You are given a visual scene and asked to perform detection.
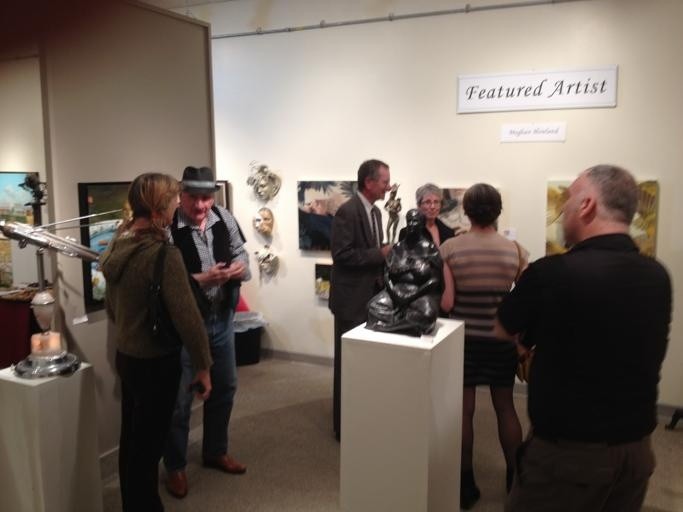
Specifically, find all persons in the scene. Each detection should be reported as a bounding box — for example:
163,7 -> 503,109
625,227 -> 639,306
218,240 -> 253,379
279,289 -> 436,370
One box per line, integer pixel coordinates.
329,159 -> 395,442
399,183 -> 457,249
299,194 -> 352,230
366,209 -> 446,337
494,166 -> 674,512
254,208 -> 273,238
163,166 -> 252,498
246,163 -> 282,200
438,184 -> 536,503
101,173 -> 215,512
254,245 -> 278,274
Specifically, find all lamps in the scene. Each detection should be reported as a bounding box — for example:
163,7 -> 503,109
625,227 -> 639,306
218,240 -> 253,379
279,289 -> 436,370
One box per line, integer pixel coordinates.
16,290 -> 80,378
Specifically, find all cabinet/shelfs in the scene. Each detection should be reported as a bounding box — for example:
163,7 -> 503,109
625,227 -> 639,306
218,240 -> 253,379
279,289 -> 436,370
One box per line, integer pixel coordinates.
340,317 -> 466,511
0,363 -> 102,512
1,288 -> 46,366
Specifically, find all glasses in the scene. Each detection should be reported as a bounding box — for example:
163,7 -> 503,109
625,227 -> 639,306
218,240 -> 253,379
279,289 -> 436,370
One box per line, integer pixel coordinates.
422,200 -> 442,205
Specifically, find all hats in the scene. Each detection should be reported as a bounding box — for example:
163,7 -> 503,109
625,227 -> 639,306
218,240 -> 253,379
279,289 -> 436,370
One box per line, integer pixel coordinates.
177,166 -> 222,194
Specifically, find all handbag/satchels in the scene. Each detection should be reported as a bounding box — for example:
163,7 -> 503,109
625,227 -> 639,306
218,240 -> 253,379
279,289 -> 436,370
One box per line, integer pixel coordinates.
148,241 -> 215,342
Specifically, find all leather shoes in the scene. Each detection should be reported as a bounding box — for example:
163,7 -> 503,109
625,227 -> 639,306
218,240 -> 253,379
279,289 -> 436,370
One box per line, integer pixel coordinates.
463,488 -> 478,511
505,471 -> 514,493
164,461 -> 190,498
202,456 -> 249,475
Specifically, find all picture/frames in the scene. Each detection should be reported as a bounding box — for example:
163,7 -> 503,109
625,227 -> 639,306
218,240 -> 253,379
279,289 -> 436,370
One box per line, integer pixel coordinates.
214,181 -> 229,211
0,172 -> 41,239
544,180 -> 660,267
78,180 -> 178,314
298,180 -> 359,251
